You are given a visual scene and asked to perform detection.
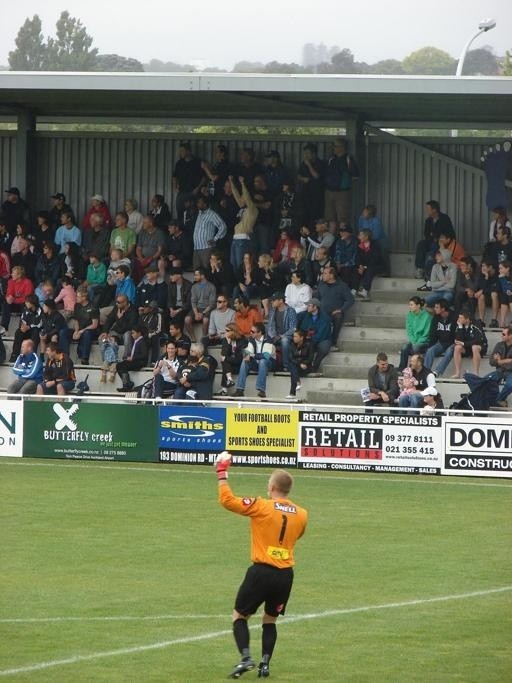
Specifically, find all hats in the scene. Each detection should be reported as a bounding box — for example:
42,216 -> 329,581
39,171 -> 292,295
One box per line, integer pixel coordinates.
263,151 -> 280,158
420,386 -> 438,395
268,291 -> 285,300
304,298 -> 321,307
91,194 -> 104,203
50,192 -> 65,200
141,300 -> 158,309
4,187 -> 20,194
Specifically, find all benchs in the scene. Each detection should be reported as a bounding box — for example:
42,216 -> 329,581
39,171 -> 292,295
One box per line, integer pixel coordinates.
0,240 -> 512,419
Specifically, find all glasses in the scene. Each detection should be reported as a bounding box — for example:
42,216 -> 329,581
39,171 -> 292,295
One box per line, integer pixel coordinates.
216,300 -> 227,303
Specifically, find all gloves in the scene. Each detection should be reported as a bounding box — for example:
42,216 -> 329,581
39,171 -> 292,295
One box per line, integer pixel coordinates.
215,451 -> 233,479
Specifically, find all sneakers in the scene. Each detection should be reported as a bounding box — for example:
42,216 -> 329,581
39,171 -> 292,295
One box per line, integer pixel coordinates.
258,662 -> 269,678
213,380 -> 244,396
417,284 -> 432,290
477,319 -> 485,327
257,390 -> 265,397
286,395 -> 296,399
350,288 -> 357,296
296,386 -> 304,390
117,381 -> 134,392
80,358 -> 88,365
229,657 -> 256,678
100,375 -> 106,382
415,268 -> 424,278
489,319 -> 499,327
109,376 -> 115,382
358,289 -> 368,297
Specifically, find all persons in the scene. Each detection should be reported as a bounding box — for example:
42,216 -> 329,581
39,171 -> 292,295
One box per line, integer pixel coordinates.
1,174 -> 385,400
171,139 -> 360,250
211,450 -> 309,679
365,199 -> 511,413
